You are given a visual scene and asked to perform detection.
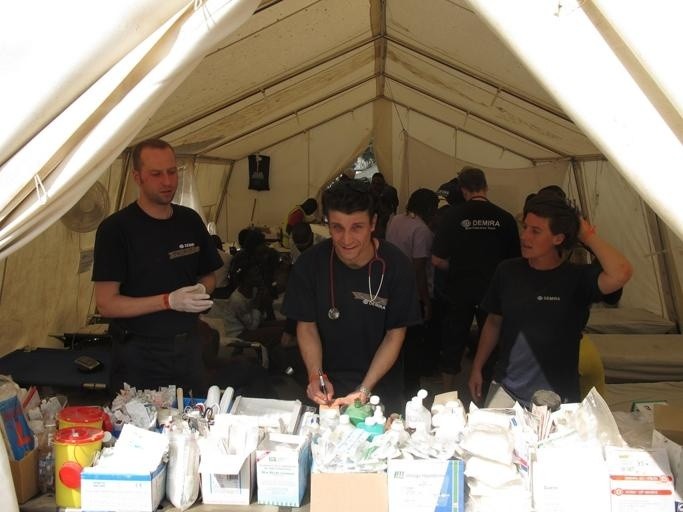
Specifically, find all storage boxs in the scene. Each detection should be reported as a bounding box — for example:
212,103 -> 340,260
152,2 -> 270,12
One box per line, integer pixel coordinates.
312,418 -> 390,512
391,393 -> 464,511
650,403 -> 683,499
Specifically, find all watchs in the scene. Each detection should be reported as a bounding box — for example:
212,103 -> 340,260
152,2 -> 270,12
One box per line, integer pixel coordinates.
357,385 -> 371,396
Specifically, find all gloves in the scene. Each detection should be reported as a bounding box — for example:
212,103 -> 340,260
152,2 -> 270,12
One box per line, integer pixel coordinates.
166,284 -> 213,313
188,283 -> 207,294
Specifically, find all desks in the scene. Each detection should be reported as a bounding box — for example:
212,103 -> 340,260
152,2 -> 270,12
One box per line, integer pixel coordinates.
30,411 -> 680,508
309,223 -> 330,244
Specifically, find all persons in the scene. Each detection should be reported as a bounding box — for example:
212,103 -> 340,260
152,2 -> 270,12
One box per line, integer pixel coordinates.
281,178 -> 422,414
239,232 -> 279,305
340,168 -> 355,182
229,228 -> 288,341
424,178 -> 466,275
209,235 -> 234,299
286,198 -> 327,253
430,168 -> 523,394
369,172 -> 399,238
91,138 -> 224,392
384,188 -> 438,401
470,193 -> 635,408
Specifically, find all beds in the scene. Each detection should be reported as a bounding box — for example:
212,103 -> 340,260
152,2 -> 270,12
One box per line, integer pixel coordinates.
587,307 -> 676,334
586,334 -> 683,380
604,380 -> 683,446
3,340 -> 263,396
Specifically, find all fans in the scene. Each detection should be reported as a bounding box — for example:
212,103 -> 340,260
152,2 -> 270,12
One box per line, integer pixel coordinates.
61,180 -> 110,252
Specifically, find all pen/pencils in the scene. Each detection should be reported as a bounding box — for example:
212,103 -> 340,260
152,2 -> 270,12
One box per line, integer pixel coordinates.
318,368 -> 327,400
